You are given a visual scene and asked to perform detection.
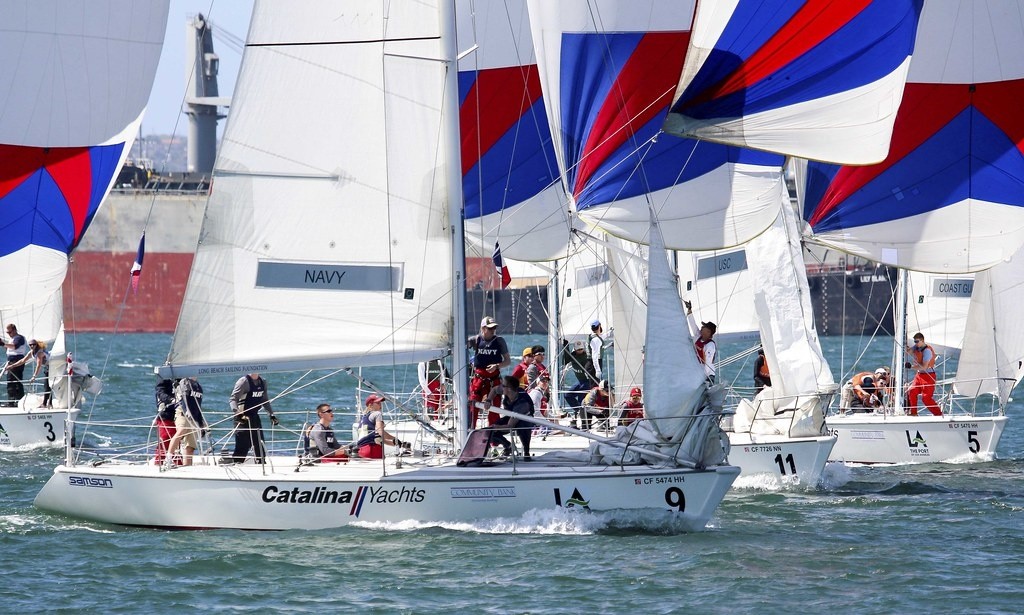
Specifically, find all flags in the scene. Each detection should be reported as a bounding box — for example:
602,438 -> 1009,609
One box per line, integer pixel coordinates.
130,233 -> 145,296
492,243 -> 512,291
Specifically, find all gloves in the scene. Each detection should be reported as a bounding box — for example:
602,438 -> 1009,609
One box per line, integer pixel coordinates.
905,362 -> 911,369
685,301 -> 692,308
270,415 -> 279,426
235,413 -> 247,425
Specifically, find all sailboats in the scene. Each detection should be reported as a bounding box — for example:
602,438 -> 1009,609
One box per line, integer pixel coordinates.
1,0 -> 1024,537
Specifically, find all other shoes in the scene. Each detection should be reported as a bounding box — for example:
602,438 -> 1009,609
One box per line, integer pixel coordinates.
499,446 -> 517,460
524,453 -> 531,462
164,460 -> 175,469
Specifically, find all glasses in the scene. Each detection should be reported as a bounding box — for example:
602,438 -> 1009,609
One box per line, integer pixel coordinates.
323,409 -> 332,414
7,331 -> 12,334
535,352 -> 545,356
29,343 -> 37,346
914,339 -> 923,343
880,382 -> 887,386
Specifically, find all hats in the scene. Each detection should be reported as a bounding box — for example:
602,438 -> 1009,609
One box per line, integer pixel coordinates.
536,376 -> 550,384
701,321 -> 716,334
574,341 -> 584,351
366,395 -> 386,407
599,379 -> 614,392
523,348 -> 532,357
481,316 -> 499,328
875,368 -> 887,374
630,388 -> 642,396
590,320 -> 599,328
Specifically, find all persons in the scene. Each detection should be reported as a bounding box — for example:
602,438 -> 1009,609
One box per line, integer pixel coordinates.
561,340 -> 591,414
466,315 -> 514,448
527,343 -> 551,414
687,302 -> 718,385
618,388 -> 643,426
6,339 -> 54,407
484,373 -> 536,462
418,360 -> 454,420
510,347 -> 533,387
527,376 -> 551,433
161,376 -> 204,466
571,379 -> 611,432
837,333 -> 944,415
586,324 -> 613,387
355,395 -> 411,458
304,401 -> 349,462
154,377 -> 183,465
0,324 -> 27,407
751,344 -> 771,392
227,373 -> 279,464
